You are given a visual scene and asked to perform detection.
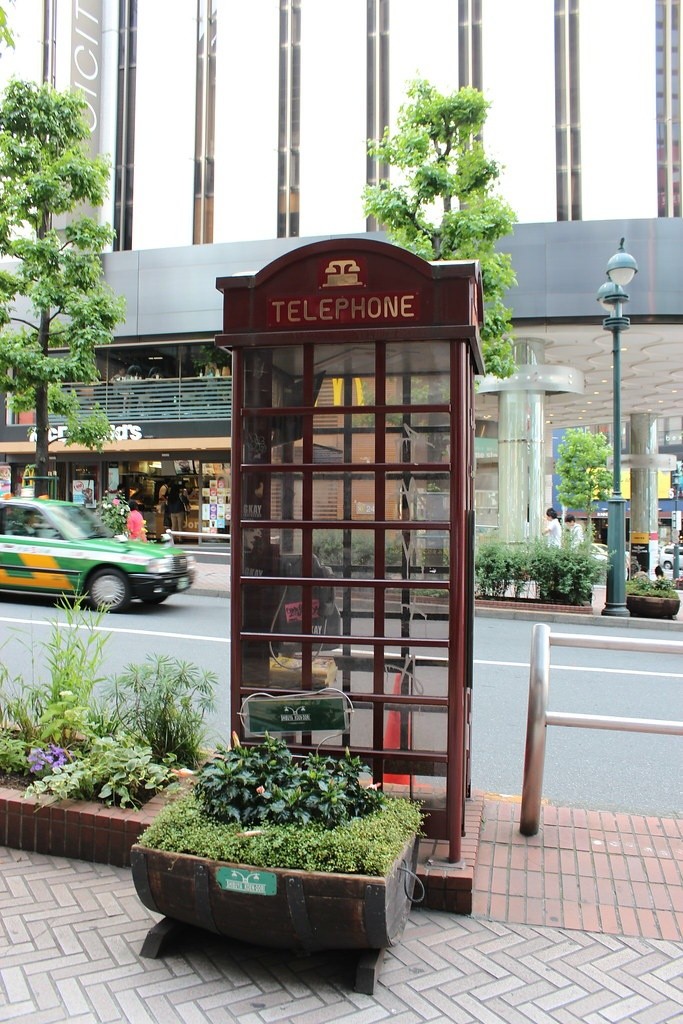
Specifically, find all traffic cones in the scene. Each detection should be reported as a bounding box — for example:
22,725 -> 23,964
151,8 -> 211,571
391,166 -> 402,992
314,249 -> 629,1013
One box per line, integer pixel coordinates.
369,673 -> 434,788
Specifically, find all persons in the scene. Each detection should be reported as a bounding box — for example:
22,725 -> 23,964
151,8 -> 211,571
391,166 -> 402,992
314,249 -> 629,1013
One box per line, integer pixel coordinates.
565,514 -> 583,550
109,367 -> 135,415
128,502 -> 145,541
18,510 -> 38,538
159,476 -> 190,532
542,508 -> 563,548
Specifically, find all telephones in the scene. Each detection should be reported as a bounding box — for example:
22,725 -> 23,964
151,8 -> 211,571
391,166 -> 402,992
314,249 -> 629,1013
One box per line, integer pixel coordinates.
272,555 -> 342,654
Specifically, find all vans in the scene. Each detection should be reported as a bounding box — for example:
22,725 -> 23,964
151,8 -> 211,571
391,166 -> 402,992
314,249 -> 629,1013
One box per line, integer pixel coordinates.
660,546 -> 683,571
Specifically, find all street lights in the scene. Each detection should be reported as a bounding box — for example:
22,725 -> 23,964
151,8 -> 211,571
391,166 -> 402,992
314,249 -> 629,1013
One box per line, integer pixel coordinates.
595,238 -> 639,617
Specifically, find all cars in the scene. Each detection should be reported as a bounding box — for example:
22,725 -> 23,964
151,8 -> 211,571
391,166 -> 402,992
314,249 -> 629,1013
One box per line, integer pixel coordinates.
0,494 -> 198,614
590,543 -> 629,564
